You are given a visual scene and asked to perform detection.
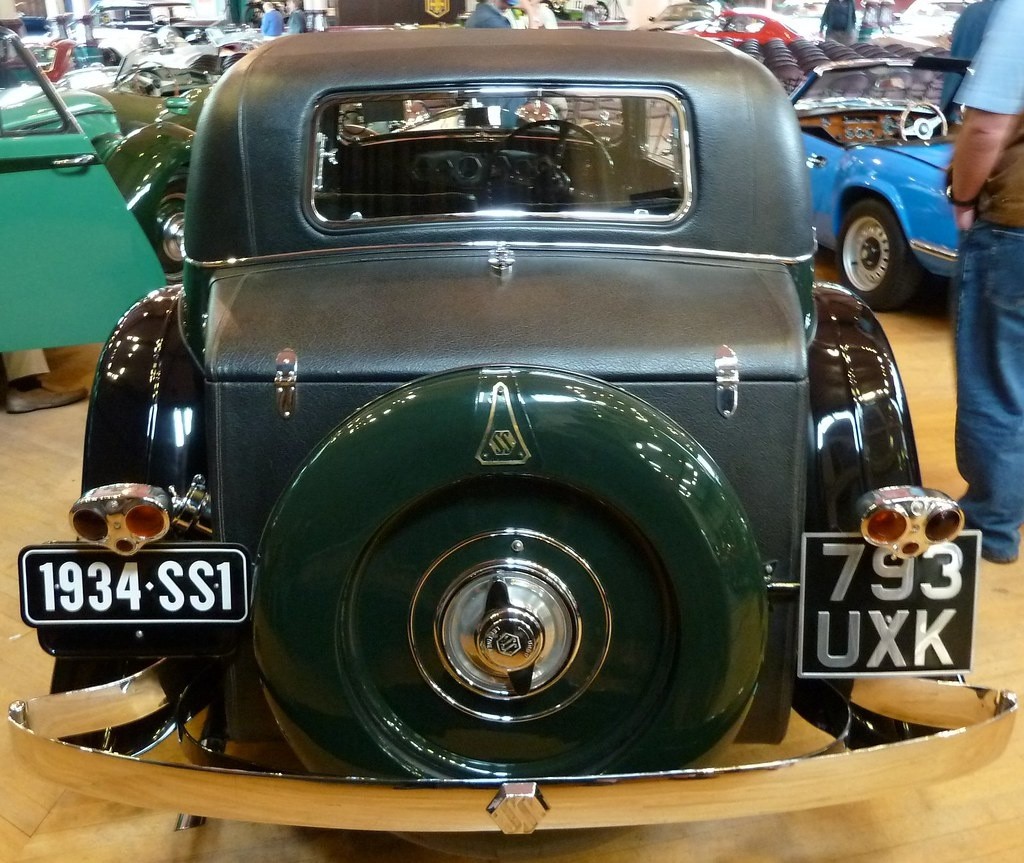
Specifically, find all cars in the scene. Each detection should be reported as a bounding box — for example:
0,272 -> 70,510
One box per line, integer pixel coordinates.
0,0 -> 1024,387
5,26 -> 1024,833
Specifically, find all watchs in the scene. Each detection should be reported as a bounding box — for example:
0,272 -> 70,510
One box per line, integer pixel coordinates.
945,185 -> 975,207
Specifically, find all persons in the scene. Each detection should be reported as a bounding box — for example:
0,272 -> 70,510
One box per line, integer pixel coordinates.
820,0 -> 857,43
262,2 -> 282,36
306,10 -> 328,31
288,0 -> 306,33
52,15 -> 68,39
465,0 -> 558,29
75,15 -> 97,47
940,0 -> 1024,564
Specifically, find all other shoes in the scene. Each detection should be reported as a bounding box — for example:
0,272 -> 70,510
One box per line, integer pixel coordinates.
7,381 -> 88,412
